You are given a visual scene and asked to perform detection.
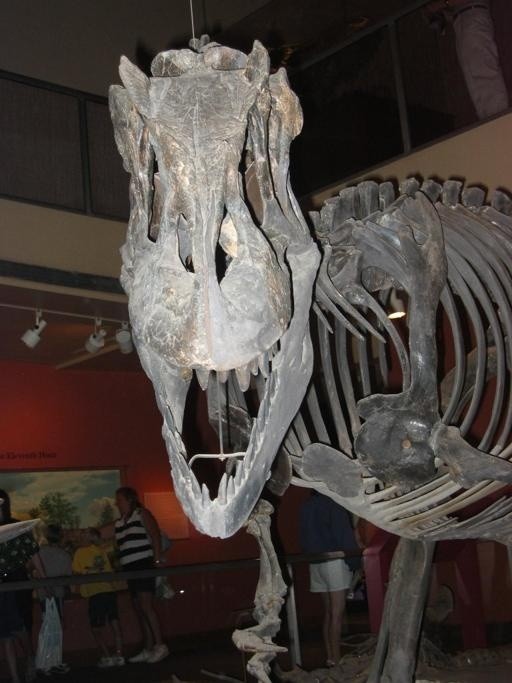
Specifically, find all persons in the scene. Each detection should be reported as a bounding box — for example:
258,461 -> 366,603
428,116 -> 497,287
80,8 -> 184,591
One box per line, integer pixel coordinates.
72,525 -> 126,668
414,0 -> 509,121
1,488 -> 55,682
298,488 -> 367,667
112,486 -> 170,663
32,524 -> 76,677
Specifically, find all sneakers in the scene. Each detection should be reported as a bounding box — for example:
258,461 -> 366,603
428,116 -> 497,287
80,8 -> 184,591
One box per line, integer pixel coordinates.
146,644 -> 170,664
41,667 -> 53,677
98,655 -> 115,668
127,648 -> 152,664
53,662 -> 69,675
112,653 -> 126,666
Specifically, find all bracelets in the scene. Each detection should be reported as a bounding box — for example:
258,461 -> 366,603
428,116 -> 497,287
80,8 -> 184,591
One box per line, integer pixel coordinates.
153,559 -> 160,564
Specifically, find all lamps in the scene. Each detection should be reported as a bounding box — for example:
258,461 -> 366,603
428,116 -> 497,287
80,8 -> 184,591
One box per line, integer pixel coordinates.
82,316 -> 135,357
16,312 -> 52,347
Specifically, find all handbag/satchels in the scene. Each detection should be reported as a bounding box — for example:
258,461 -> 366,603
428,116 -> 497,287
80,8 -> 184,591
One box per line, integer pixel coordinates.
34,596 -> 63,671
140,506 -> 171,552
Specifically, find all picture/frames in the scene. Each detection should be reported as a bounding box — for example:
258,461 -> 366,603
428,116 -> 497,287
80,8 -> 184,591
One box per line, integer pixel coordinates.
0,464 -> 131,602
